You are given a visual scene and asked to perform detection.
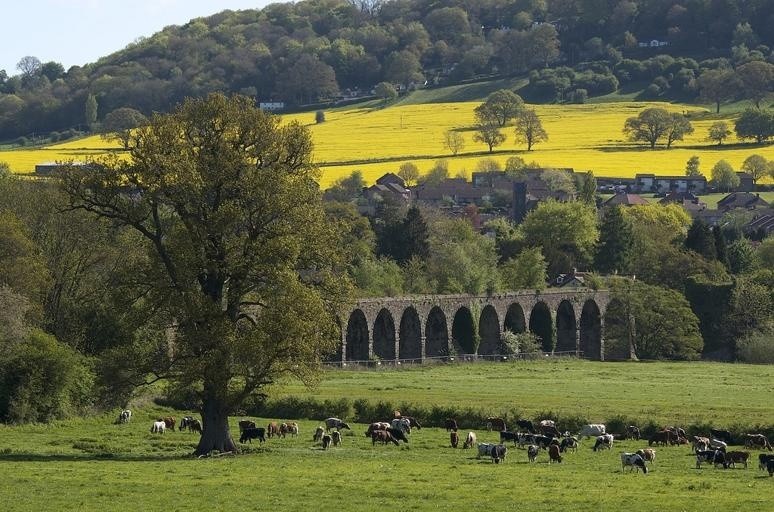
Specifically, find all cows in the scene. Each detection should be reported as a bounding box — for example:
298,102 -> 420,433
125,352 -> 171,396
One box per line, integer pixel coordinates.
445,418 -> 615,464
625,424 -> 640,441
649,426 -> 689,447
313,418 -> 350,450
152,416 -> 202,434
364,408 -> 421,446
619,448 -> 657,474
240,421 -> 299,447
117,410 -> 131,424
692,431 -> 774,476
